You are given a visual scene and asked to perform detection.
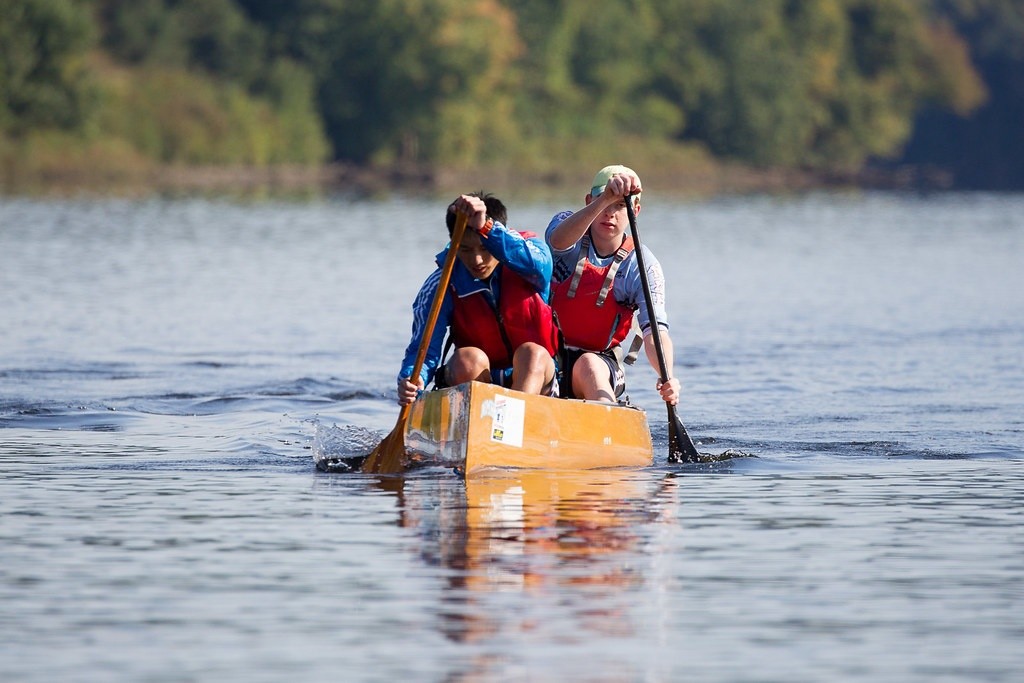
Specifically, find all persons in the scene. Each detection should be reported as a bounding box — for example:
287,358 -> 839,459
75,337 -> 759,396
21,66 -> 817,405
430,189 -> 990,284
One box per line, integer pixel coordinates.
545,165 -> 681,407
398,189 -> 562,399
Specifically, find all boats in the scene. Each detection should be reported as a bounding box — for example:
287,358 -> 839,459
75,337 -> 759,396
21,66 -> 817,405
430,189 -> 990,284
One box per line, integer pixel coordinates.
361,379 -> 654,475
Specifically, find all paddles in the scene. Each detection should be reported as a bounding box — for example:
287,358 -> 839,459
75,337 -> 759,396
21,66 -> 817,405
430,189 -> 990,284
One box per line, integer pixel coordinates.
361,211 -> 470,474
615,184 -> 699,463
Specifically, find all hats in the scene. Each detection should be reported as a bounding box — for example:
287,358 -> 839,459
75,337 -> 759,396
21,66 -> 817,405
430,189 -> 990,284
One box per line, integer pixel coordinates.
591,165 -> 643,202
